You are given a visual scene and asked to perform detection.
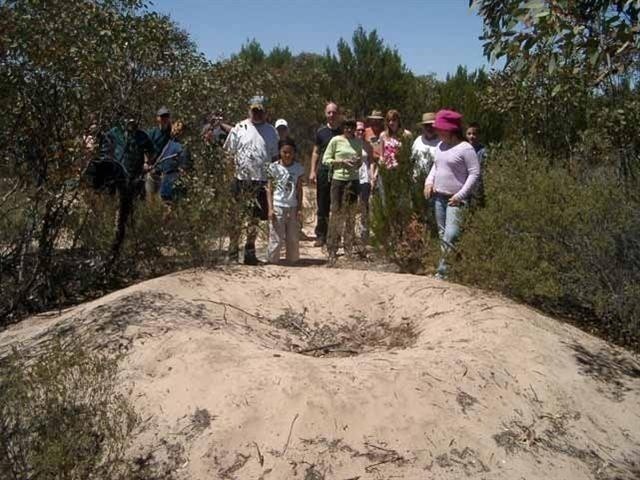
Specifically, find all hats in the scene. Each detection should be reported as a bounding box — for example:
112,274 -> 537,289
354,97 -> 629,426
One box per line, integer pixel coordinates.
432,109 -> 464,131
247,96 -> 271,112
275,119 -> 288,128
416,112 -> 436,125
342,120 -> 356,128
368,110 -> 384,119
157,106 -> 170,116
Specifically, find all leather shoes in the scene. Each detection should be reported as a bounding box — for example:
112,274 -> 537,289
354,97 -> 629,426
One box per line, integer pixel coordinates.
314,240 -> 325,247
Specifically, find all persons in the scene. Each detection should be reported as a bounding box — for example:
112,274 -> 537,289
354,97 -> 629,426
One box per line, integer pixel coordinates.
144,105 -> 172,201
199,109 -> 236,147
411,111 -> 439,239
220,94 -> 280,266
422,109 -> 481,280
306,100 -> 411,261
100,115 -> 153,203
265,139 -> 306,266
464,121 -> 488,208
158,120 -> 191,208
272,118 -> 297,162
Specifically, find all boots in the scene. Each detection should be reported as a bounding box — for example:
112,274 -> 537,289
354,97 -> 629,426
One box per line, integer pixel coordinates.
244,244 -> 264,265
